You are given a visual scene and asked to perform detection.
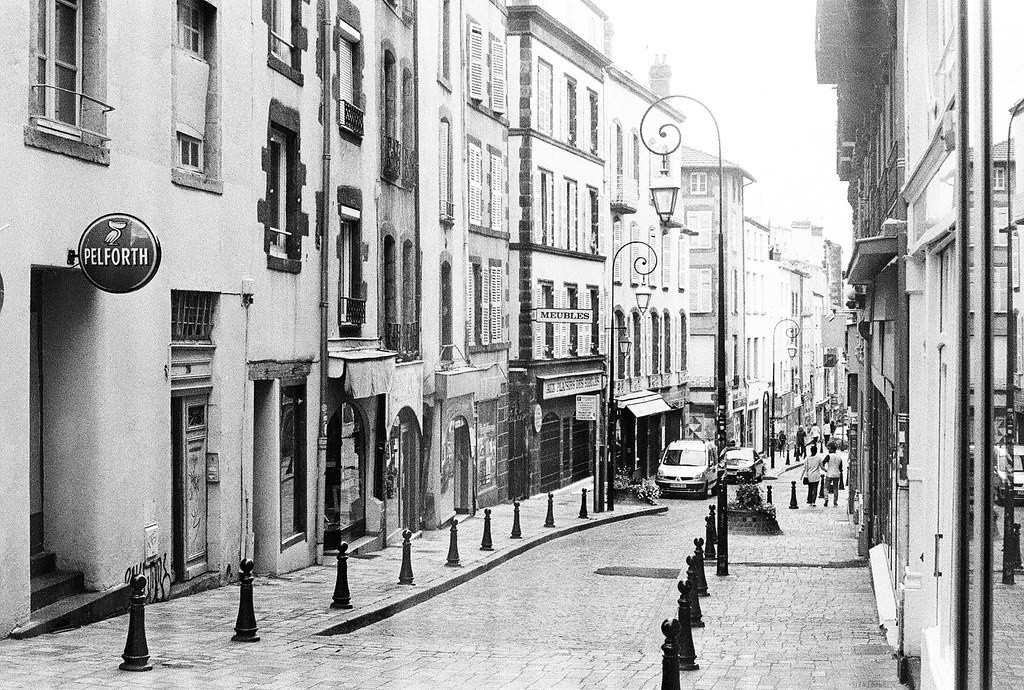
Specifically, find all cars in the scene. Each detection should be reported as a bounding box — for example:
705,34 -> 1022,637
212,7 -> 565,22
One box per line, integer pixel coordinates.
832,427 -> 849,446
721,449 -> 766,484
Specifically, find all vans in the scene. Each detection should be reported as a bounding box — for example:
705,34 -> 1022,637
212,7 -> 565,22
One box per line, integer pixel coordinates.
967,444 -> 1024,508
655,437 -> 720,500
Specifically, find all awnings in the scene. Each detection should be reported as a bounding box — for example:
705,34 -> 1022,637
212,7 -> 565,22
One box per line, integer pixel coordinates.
619,391 -> 672,418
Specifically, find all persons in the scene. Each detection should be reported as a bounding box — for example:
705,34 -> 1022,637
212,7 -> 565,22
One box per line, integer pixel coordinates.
810,423 -> 821,447
830,419 -> 842,434
824,421 -> 830,444
823,445 -> 843,507
797,427 -> 806,456
800,446 -> 827,506
778,430 -> 787,457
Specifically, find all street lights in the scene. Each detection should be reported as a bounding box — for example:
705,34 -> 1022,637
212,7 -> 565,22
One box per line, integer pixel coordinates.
607,240 -> 659,513
639,93 -> 732,578
771,319 -> 801,469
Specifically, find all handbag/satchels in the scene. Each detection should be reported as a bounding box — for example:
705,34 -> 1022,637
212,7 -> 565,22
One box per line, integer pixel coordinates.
802,457 -> 809,485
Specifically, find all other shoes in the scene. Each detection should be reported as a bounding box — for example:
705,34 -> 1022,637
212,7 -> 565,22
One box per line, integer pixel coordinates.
809,503 -> 812,506
833,502 -> 838,506
811,502 -> 815,507
824,499 -> 829,507
